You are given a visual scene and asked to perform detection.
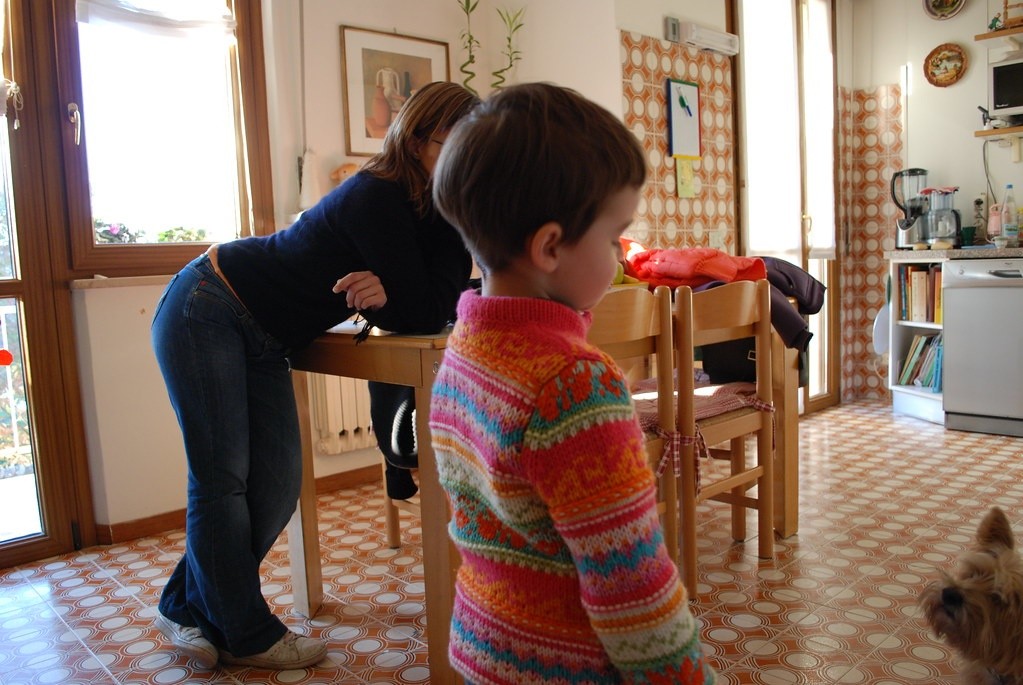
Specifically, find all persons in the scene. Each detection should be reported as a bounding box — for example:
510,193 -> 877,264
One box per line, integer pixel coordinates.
427,82 -> 732,685
149,81 -> 487,669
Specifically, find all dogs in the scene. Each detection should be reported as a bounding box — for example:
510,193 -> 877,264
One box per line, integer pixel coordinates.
915,501 -> 1023,685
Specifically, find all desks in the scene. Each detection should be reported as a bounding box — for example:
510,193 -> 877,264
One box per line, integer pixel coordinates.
291,293 -> 802,619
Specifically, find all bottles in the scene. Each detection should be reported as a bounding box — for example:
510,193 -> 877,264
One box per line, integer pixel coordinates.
1002,184 -> 1018,248
1018,208 -> 1023,242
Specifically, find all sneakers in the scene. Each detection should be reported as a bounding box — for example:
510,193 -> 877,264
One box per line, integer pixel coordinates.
153,614 -> 218,669
219,629 -> 326,670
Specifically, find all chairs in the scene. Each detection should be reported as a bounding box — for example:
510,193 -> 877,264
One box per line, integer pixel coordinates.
581,278 -> 775,599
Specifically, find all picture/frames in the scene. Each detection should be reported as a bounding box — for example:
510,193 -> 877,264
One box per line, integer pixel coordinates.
341,24 -> 451,158
667,78 -> 701,160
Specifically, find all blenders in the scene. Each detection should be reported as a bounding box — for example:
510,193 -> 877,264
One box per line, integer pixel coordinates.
890,168 -> 962,250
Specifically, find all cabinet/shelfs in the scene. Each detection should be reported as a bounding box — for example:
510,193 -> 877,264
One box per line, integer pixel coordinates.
974,26 -> 1023,141
888,260 -> 944,426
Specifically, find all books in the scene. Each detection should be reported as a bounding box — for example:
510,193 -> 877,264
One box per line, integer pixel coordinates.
898,263 -> 942,325
897,333 -> 942,391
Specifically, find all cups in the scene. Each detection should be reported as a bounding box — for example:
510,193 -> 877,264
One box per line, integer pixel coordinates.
991,237 -> 1008,249
962,227 -> 976,246
